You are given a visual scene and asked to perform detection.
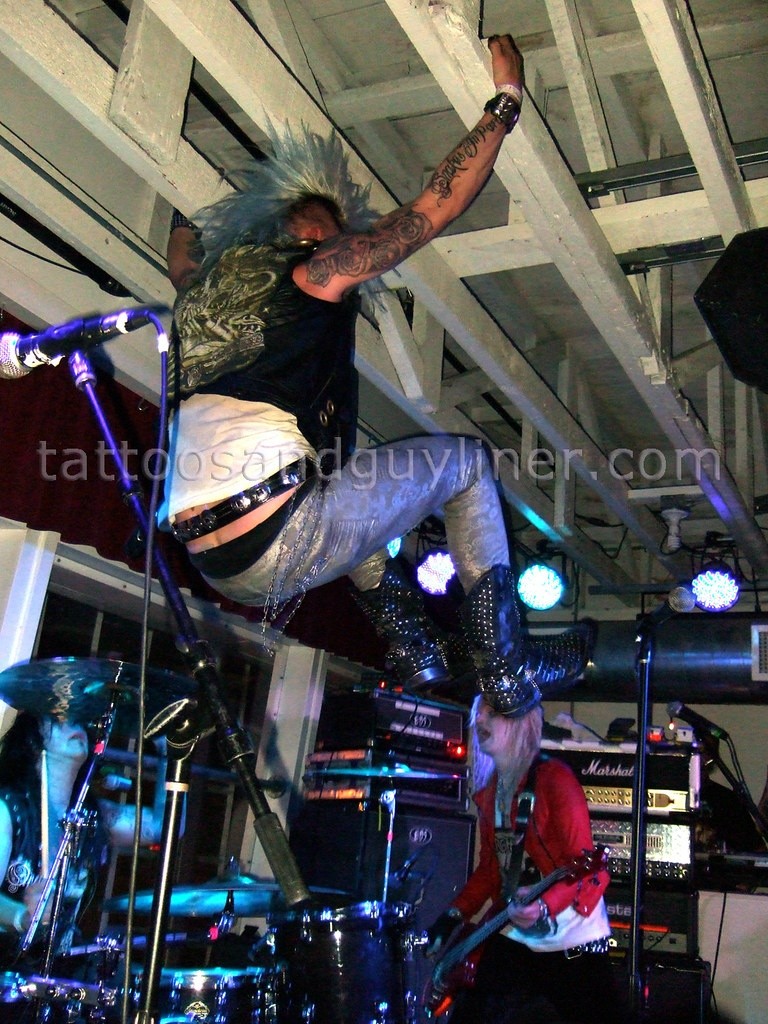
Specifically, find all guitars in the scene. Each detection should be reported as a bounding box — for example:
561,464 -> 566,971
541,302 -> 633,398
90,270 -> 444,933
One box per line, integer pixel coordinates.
419,842 -> 605,1024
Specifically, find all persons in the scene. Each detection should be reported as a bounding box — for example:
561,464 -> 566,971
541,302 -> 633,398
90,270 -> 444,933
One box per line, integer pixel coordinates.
161,33 -> 597,718
0,711 -> 186,976
424,695 -> 611,1024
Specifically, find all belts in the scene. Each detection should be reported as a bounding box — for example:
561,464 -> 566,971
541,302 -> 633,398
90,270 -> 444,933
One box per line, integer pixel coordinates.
171,457 -> 323,544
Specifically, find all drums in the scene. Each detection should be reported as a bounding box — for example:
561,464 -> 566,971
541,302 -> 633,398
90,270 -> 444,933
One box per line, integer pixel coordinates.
125,967 -> 273,1024
266,901 -> 419,1024
0,970 -> 141,1024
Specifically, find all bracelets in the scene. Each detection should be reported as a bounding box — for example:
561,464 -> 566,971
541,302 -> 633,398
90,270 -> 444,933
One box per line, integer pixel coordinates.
484,93 -> 521,135
169,209 -> 203,240
538,898 -> 548,925
444,908 -> 463,921
14,909 -> 27,933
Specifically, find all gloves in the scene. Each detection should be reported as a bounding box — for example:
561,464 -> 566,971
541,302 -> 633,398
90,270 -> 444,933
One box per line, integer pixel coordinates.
424,913 -> 461,958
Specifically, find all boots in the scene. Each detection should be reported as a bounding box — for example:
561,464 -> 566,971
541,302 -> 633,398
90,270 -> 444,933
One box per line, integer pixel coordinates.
349,559 -> 461,690
455,565 -> 598,718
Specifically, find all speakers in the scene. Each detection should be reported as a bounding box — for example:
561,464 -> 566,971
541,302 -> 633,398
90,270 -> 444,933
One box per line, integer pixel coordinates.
298,795 -> 477,1024
611,954 -> 713,1024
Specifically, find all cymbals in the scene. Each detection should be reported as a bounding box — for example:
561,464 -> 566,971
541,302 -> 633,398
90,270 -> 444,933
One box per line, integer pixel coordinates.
98,874 -> 361,918
1,656 -> 198,739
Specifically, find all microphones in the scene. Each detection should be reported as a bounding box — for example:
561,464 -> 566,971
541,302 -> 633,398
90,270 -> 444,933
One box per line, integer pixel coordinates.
0,310 -> 152,379
638,587 -> 695,635
665,701 -> 729,740
